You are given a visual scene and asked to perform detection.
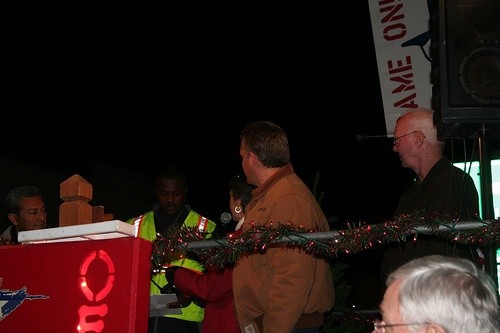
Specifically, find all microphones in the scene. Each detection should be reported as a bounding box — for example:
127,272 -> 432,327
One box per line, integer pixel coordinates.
213,212 -> 232,240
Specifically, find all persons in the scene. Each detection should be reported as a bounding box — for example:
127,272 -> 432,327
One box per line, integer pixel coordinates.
230,120 -> 338,333
165,180 -> 259,333
125,172 -> 221,333
375,255 -> 500,333
2,183 -> 48,243
391,109 -> 482,268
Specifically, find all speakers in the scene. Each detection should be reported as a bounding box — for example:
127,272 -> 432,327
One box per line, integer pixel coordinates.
431,0 -> 500,125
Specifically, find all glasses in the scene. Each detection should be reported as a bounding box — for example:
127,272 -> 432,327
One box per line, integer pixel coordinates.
373,319 -> 431,333
393,130 -> 425,146
